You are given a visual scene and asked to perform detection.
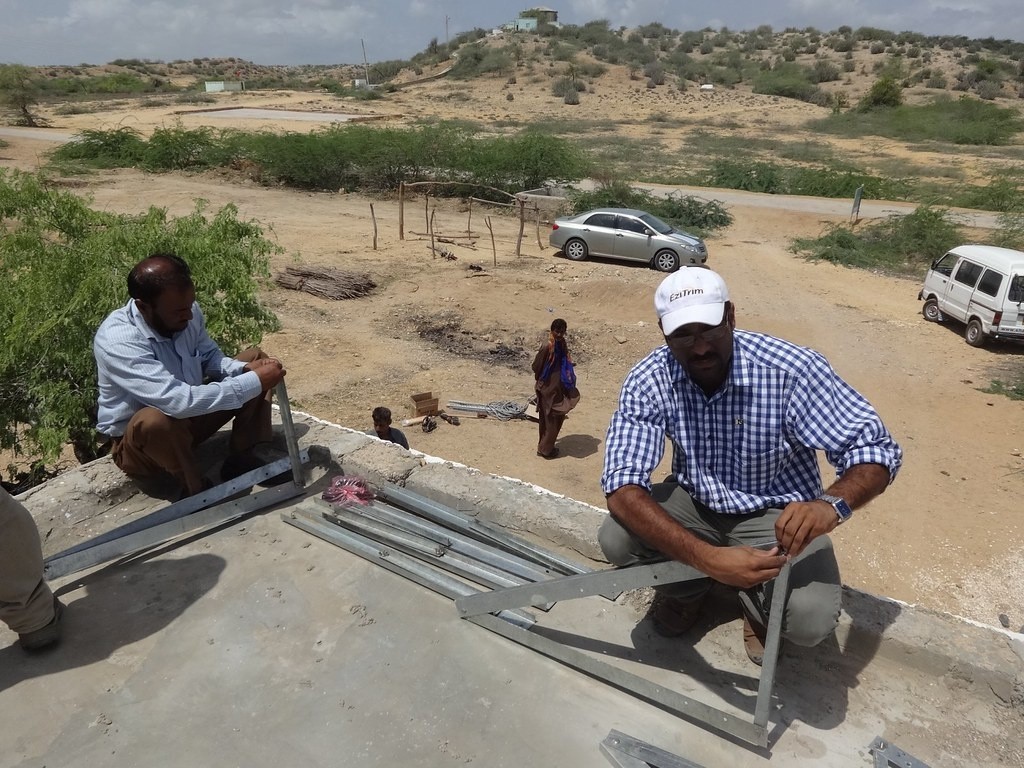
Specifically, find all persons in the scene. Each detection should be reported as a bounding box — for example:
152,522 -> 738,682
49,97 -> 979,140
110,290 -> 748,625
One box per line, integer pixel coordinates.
0,486 -> 63,656
91,253 -> 286,501
598,265 -> 903,666
362,406 -> 410,451
531,318 -> 577,460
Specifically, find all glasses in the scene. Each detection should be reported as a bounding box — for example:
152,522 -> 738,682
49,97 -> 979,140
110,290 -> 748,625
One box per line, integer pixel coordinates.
666,311 -> 729,350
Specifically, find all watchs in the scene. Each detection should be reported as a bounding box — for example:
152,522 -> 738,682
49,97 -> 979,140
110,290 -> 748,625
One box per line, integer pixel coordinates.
816,494 -> 852,526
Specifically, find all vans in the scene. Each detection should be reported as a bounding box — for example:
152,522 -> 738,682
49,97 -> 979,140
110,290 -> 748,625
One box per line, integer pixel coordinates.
919,247 -> 1024,348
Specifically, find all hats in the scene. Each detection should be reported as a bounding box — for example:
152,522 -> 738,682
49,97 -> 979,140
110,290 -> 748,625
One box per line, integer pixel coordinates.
654,266 -> 730,336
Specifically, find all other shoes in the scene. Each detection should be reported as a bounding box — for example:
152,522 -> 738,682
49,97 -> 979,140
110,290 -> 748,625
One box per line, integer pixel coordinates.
744,611 -> 785,666
537,448 -> 559,456
221,457 -> 291,486
655,596 -> 703,637
19,594 -> 64,653
539,449 -> 558,459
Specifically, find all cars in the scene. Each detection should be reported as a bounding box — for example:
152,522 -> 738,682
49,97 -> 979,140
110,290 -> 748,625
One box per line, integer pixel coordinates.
548,209 -> 708,273
